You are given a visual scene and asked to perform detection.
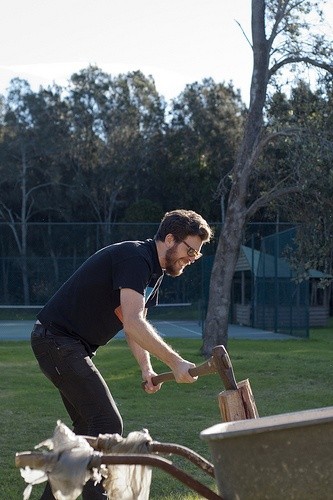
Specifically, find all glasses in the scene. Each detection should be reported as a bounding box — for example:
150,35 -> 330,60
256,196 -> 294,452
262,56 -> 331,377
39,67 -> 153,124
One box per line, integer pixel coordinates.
173,233 -> 203,260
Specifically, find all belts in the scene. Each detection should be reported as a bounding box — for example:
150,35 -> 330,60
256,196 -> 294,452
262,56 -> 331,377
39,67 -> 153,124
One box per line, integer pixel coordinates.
32,324 -> 56,336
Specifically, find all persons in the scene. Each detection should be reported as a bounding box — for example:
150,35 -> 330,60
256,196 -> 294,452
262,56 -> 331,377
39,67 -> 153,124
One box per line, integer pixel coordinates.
29,208 -> 216,500
